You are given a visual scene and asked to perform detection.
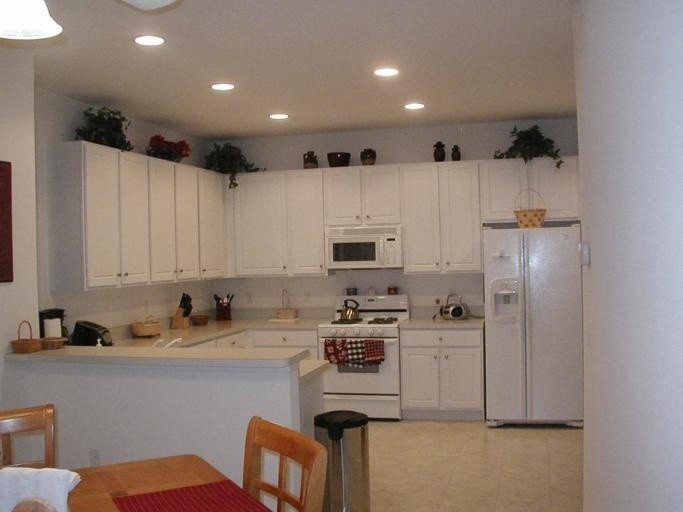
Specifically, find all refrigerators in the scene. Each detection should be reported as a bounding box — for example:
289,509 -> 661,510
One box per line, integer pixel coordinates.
483,224 -> 583,426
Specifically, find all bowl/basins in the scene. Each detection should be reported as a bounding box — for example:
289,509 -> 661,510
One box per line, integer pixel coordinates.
190,315 -> 209,327
327,152 -> 351,166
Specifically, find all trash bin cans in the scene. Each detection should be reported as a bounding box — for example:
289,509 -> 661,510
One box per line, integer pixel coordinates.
312,410 -> 369,512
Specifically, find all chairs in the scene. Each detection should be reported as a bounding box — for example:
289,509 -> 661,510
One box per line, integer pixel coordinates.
0,404 -> 64,469
243,416 -> 332,511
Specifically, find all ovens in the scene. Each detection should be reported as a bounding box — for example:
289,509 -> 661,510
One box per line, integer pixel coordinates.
318,338 -> 400,397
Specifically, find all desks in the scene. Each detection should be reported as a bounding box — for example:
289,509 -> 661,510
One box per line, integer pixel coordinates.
60,454 -> 273,511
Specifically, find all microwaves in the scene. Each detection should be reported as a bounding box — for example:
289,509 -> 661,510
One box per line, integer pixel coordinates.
324,226 -> 402,270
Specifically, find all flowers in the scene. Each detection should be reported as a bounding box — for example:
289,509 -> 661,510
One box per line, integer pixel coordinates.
147,133 -> 193,162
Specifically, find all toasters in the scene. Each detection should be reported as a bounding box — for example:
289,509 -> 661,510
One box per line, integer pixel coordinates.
72,319 -> 113,345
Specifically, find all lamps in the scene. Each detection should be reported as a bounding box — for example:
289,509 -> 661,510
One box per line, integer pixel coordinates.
1,1 -> 66,41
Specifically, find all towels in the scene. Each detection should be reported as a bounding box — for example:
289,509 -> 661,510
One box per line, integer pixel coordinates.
323,336 -> 349,366
0,466 -> 83,511
363,338 -> 385,366
341,340 -> 367,370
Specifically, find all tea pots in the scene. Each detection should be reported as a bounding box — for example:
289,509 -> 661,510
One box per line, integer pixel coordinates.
341,299 -> 360,322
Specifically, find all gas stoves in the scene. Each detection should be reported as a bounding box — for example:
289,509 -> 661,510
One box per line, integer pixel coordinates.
331,316 -> 398,325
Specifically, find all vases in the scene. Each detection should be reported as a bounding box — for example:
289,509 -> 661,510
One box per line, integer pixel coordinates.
145,152 -> 185,163
325,152 -> 353,167
361,148 -> 376,167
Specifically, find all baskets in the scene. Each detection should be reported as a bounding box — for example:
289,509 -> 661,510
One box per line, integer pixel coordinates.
9,321 -> 42,353
131,321 -> 160,336
513,189 -> 546,228
42,336 -> 68,349
278,289 -> 297,319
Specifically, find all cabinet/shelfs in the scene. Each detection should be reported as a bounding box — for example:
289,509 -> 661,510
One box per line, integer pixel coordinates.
320,323 -> 404,425
231,170 -> 285,281
481,156 -> 579,223
175,327 -> 249,351
249,326 -> 319,351
284,167 -> 324,277
326,168 -> 399,225
148,157 -> 199,287
40,139 -> 149,293
399,160 -> 483,276
198,167 -> 233,285
400,318 -> 487,423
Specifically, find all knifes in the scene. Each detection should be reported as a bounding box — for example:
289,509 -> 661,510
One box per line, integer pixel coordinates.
179,293 -> 193,318
